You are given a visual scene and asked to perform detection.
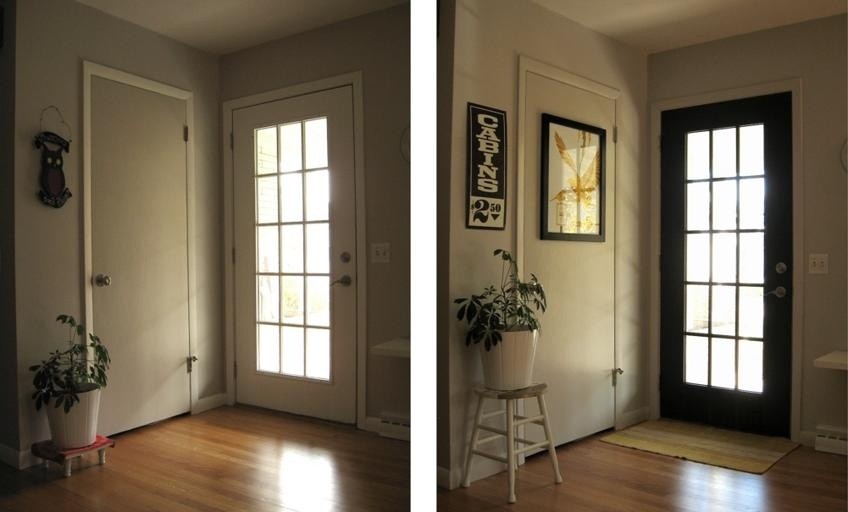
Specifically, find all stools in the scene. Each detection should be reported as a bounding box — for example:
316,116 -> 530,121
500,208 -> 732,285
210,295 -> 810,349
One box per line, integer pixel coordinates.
460,385 -> 563,505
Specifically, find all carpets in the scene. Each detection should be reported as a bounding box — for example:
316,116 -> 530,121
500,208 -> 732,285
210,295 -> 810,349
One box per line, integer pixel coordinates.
596,416 -> 802,475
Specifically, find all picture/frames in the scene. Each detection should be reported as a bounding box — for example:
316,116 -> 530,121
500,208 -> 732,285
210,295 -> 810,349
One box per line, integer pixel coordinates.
537,109 -> 607,243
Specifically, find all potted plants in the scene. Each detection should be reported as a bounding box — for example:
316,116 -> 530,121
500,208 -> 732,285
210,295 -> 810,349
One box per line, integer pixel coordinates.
450,246 -> 549,392
25,312 -> 112,448
